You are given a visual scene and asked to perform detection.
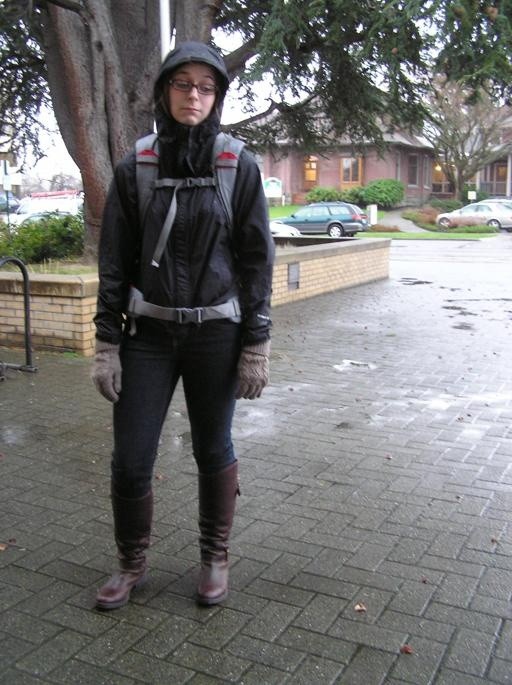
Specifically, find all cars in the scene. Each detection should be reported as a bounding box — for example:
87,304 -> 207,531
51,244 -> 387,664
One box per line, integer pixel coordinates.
270,220 -> 302,237
479,198 -> 512,233
0,192 -> 87,232
436,202 -> 511,231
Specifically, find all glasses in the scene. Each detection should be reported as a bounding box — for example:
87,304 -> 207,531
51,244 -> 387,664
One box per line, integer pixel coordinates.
168,79 -> 219,95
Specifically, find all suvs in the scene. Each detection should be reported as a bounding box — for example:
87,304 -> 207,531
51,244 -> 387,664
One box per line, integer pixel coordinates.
272,199 -> 370,238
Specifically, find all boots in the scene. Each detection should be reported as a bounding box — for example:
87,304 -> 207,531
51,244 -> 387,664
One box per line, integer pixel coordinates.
197,458 -> 240,606
96,480 -> 153,609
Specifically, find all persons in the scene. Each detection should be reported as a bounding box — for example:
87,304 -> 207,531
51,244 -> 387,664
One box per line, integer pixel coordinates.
88,38 -> 280,614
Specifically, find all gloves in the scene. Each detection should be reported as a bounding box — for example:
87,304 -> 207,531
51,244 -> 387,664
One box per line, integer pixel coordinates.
235,339 -> 271,400
92,338 -> 123,404
